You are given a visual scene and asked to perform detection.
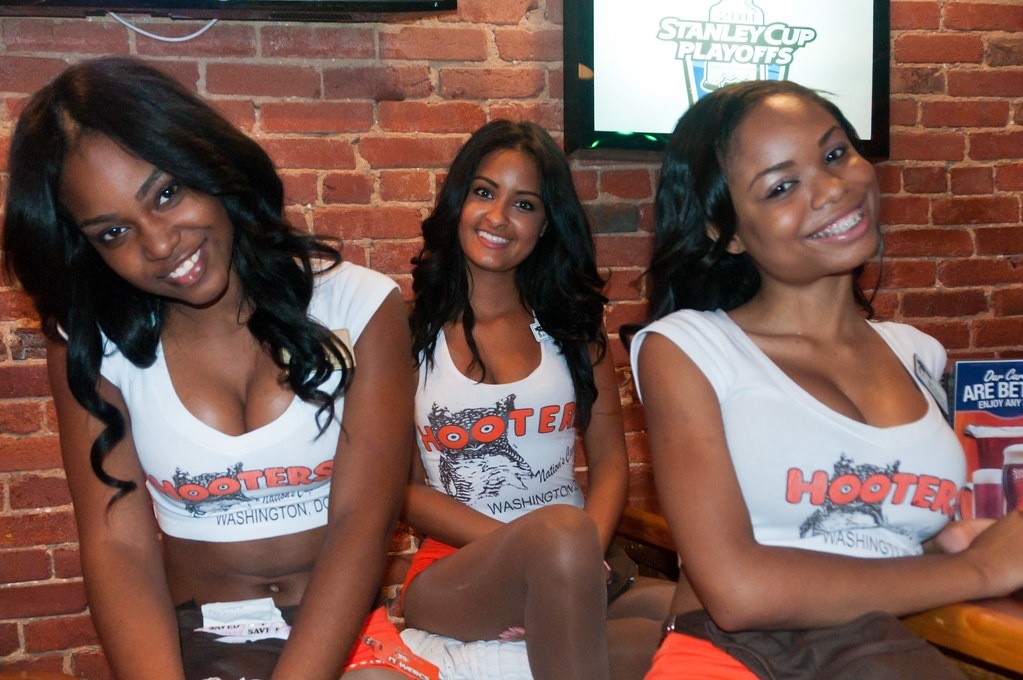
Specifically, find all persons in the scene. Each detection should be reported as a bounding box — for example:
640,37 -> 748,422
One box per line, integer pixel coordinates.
624,79 -> 1023,680
404,117 -> 675,680
0,56 -> 421,680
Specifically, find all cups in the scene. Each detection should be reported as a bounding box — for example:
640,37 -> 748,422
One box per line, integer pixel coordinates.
954,468 -> 1005,521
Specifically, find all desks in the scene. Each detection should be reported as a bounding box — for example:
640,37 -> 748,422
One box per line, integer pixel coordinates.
617,503 -> 1023,680
